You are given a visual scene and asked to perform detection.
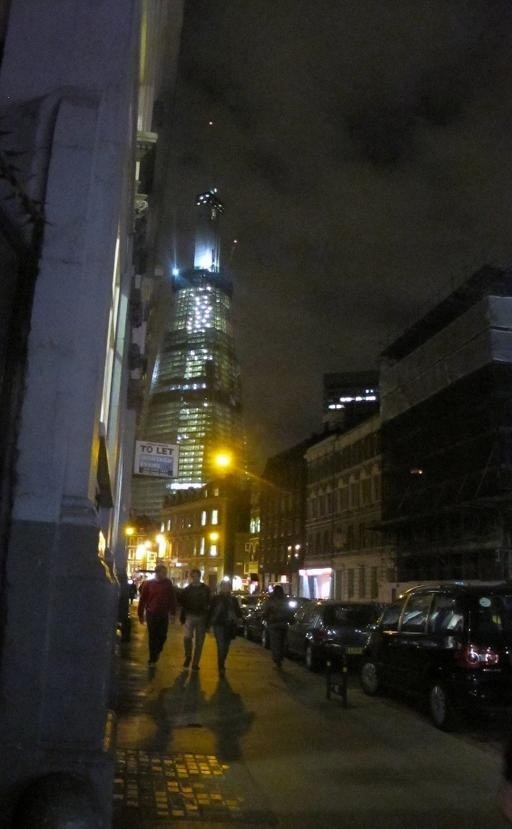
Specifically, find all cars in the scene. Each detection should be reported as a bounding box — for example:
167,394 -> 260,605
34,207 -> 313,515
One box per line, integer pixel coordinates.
287,599 -> 382,671
227,590 -> 312,648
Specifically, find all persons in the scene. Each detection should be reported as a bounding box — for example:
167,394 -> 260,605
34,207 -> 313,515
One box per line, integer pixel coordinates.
210,580 -> 245,678
137,563 -> 175,669
256,584 -> 294,670
176,568 -> 212,670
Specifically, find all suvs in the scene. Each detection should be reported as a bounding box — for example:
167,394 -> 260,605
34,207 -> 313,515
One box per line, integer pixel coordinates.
357,582 -> 510,733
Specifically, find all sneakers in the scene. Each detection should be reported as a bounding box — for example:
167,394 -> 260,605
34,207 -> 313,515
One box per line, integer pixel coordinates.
184,657 -> 201,671
